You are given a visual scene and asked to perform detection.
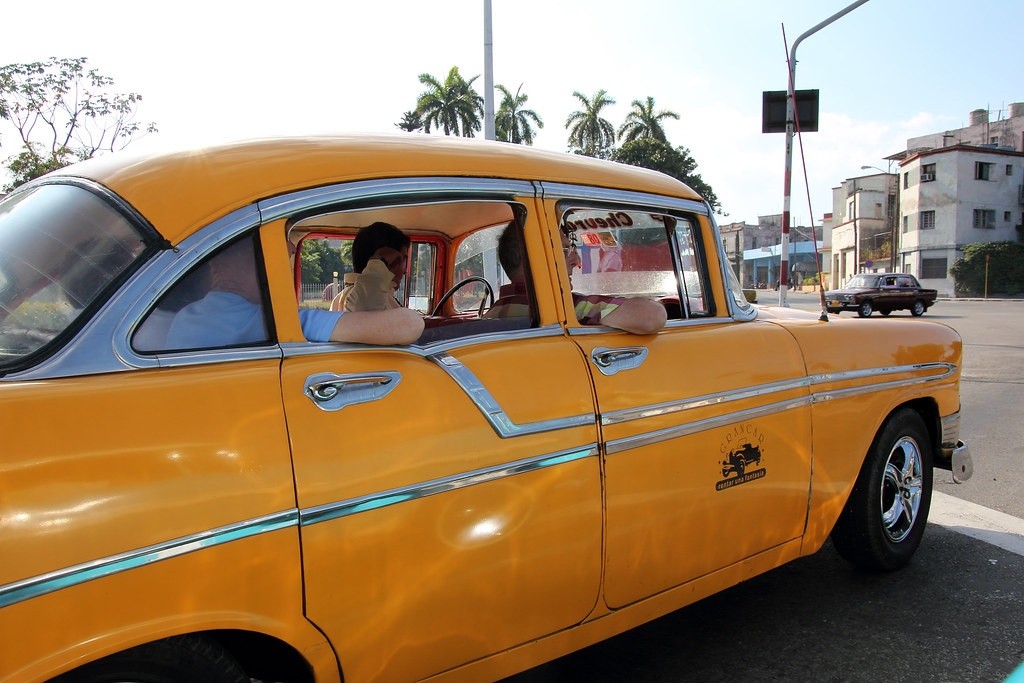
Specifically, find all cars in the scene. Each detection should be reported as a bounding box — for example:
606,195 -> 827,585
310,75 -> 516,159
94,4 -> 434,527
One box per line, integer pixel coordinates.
1,134 -> 973,683
823,273 -> 938,317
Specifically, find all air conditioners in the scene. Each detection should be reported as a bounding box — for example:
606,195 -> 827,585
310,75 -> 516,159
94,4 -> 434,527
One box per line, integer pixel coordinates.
920,174 -> 932,181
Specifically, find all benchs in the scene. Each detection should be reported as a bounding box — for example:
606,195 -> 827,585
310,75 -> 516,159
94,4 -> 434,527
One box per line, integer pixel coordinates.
417,315 -> 531,346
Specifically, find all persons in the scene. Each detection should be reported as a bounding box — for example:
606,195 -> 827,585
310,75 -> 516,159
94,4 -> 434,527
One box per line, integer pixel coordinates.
61,219 -> 425,352
330,222 -> 411,310
480,217 -> 667,335
